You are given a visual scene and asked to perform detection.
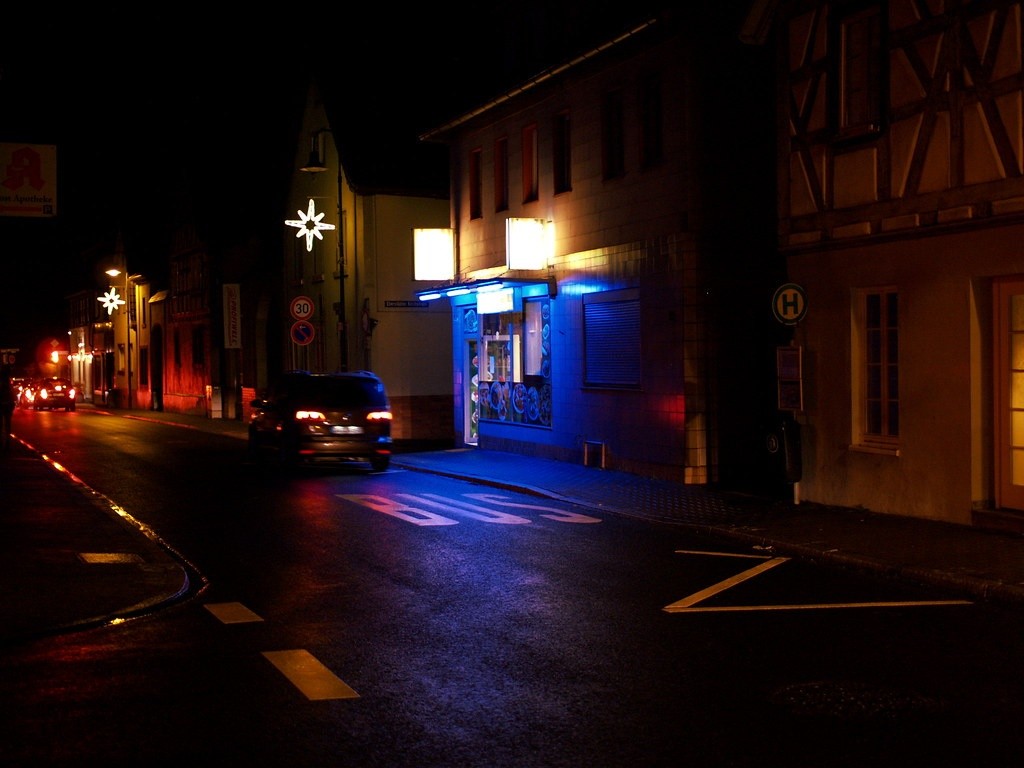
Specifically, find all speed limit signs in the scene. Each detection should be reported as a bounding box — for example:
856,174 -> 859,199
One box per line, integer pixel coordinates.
290,296 -> 315,321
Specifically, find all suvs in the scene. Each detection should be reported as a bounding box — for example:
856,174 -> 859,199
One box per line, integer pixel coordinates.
247,369 -> 394,476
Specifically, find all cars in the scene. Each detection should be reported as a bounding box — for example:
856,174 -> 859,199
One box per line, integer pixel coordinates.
10,378 -> 77,411
